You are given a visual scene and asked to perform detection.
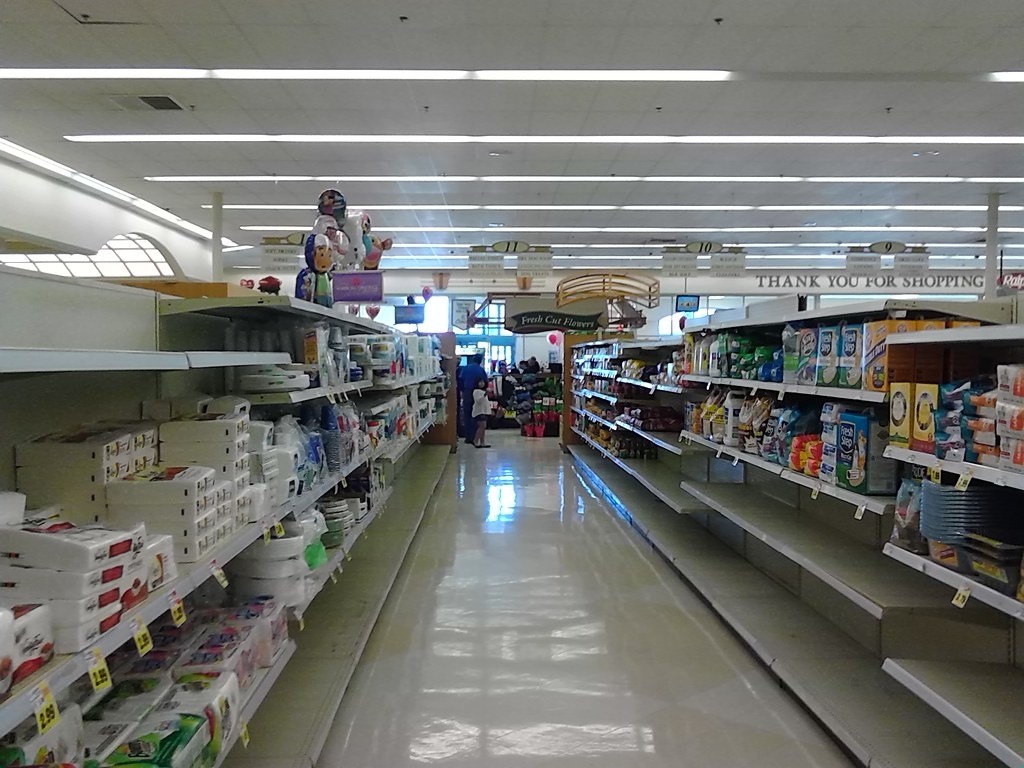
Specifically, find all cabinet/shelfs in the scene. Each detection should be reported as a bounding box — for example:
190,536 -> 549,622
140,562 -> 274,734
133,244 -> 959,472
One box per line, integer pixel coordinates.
157,293 -> 447,622
674,298 -> 1013,666
881,323 -> 1024,768
570,338 -> 746,534
0,265 -> 296,768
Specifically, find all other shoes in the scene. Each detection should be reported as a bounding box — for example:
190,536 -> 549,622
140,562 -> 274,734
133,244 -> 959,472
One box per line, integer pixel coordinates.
471,440 -> 480,448
464,439 -> 472,443
480,444 -> 491,448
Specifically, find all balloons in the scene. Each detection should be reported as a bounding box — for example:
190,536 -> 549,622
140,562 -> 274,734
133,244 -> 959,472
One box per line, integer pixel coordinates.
547,330 -> 563,348
238,187 -> 395,324
422,286 -> 433,304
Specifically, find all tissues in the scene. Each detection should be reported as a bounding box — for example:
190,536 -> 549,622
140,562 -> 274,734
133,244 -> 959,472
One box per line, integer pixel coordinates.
0,391 -> 287,768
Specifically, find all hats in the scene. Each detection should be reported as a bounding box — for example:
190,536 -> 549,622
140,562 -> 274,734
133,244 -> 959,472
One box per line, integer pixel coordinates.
473,354 -> 482,362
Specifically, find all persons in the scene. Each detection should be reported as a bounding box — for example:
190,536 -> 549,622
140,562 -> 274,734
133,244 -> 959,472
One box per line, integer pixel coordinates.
529,356 -> 539,374
498,360 -> 508,373
457,353 -> 488,444
456,355 -> 461,437
519,361 -> 536,374
471,378 -> 491,448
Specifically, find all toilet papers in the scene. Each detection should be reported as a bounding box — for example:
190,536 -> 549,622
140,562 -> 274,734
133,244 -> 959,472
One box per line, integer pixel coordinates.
224,320 -> 450,609
